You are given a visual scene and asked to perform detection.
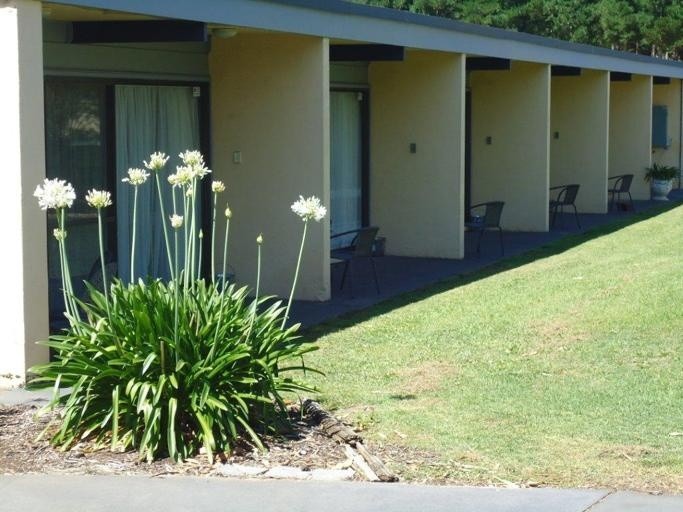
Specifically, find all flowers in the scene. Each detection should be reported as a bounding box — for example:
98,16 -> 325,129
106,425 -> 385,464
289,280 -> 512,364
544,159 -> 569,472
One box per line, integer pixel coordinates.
23,147 -> 327,465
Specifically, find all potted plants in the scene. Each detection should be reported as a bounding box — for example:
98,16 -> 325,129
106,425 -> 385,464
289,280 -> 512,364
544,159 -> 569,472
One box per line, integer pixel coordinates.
641,163 -> 682,202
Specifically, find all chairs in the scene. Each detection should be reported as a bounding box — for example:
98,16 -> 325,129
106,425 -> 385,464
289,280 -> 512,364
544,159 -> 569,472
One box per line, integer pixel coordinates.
464,201 -> 507,258
608,173 -> 634,211
549,184 -> 582,230
330,225 -> 381,295
